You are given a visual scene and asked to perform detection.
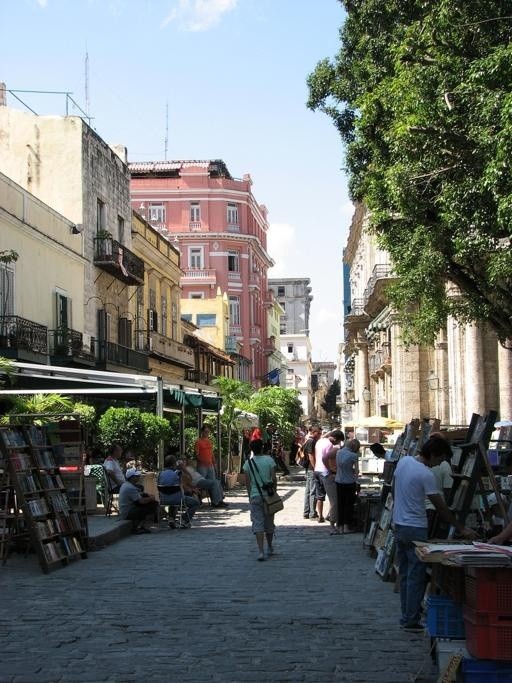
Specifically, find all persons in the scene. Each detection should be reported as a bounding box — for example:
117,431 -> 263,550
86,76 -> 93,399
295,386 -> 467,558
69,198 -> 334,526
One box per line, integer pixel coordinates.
392,434 -> 480,633
194,426 -> 216,479
118,468 -> 158,534
182,453 -> 229,508
102,445 -> 144,493
156,454 -> 200,529
265,421 -> 360,535
242,439 -> 278,561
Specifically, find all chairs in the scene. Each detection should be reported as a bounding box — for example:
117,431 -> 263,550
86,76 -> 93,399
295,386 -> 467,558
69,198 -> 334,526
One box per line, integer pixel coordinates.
156,484 -> 191,532
99,463 -> 121,517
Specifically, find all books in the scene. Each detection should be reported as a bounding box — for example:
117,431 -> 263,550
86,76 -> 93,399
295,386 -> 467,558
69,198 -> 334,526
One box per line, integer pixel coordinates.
0,427 -> 83,563
366,421 -> 512,577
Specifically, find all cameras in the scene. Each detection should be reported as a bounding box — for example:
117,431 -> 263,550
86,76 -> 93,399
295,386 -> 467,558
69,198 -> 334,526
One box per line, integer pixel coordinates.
262,483 -> 275,496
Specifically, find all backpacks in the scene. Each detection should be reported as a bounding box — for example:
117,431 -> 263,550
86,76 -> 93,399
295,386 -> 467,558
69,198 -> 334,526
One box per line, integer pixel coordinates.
293,438 -> 312,469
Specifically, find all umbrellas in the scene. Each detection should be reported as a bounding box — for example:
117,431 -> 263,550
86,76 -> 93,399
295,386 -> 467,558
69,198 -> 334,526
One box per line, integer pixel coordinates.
342,414 -> 405,444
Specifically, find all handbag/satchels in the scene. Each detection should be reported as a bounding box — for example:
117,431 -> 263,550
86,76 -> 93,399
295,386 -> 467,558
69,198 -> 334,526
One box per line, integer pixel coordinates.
262,492 -> 284,518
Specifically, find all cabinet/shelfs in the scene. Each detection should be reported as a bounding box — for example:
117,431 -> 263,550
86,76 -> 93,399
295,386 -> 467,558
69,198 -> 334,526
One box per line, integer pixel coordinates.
0,419 -> 87,575
346,409 -> 512,615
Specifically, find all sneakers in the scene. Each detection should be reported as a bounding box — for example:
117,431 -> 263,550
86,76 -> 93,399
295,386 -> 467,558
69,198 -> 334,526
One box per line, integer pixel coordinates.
255,547 -> 274,561
399,621 -> 427,633
211,501 -> 227,507
134,527 -> 152,535
302,512 -> 356,535
168,518 -> 193,529
282,470 -> 290,476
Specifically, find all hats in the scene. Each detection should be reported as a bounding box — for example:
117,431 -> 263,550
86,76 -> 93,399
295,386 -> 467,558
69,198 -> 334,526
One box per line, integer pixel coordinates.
125,467 -> 142,479
